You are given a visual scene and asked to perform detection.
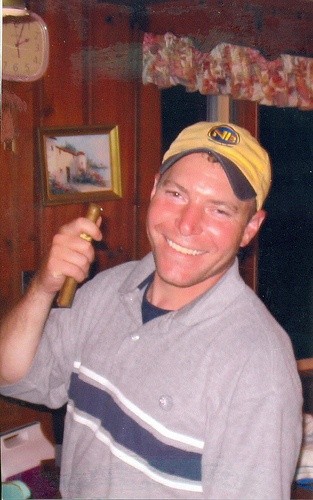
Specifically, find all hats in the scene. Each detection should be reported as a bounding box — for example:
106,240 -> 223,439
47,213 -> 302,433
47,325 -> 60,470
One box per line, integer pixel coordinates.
159,122 -> 274,212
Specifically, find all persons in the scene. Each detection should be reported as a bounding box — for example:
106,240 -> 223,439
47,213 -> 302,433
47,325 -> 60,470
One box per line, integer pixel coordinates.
0,120 -> 306,500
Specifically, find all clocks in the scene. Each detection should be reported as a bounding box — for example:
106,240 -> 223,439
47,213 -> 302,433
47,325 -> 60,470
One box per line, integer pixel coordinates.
2,7 -> 48,81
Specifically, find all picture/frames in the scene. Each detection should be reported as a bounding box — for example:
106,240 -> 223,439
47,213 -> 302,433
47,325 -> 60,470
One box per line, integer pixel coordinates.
34,124 -> 122,206
